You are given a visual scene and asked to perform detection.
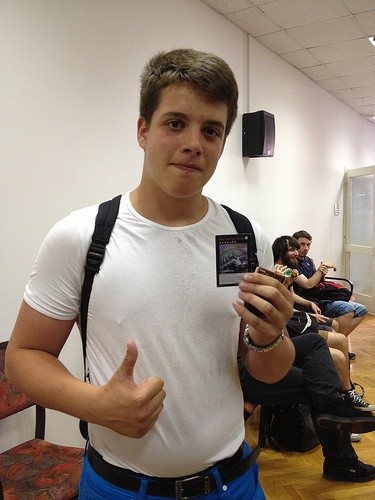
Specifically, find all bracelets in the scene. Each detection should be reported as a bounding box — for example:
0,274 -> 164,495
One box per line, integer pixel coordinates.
242,322 -> 286,354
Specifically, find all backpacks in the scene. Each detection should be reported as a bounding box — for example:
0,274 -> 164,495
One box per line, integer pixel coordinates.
302,280 -> 352,302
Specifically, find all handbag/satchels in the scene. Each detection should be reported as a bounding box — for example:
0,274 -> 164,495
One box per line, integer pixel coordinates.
266,399 -> 320,454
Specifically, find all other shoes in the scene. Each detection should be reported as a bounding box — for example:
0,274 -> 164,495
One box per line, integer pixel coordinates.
348,352 -> 356,360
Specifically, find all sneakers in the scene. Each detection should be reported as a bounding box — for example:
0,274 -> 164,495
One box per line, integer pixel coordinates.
349,433 -> 360,441
323,456 -> 375,483
317,393 -> 375,433
344,380 -> 375,411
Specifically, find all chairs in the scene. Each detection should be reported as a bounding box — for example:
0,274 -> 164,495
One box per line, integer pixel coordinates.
0,340 -> 85,500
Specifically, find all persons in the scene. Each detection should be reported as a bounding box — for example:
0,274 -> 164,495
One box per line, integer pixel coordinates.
4,49 -> 295,500
237,231 -> 375,483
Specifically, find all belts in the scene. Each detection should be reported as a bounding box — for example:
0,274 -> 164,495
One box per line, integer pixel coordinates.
87,442 -> 262,500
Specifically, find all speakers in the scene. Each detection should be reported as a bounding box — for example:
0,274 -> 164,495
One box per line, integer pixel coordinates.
242,110 -> 275,158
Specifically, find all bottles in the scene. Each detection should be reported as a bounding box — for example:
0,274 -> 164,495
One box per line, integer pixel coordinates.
279,265 -> 292,278
